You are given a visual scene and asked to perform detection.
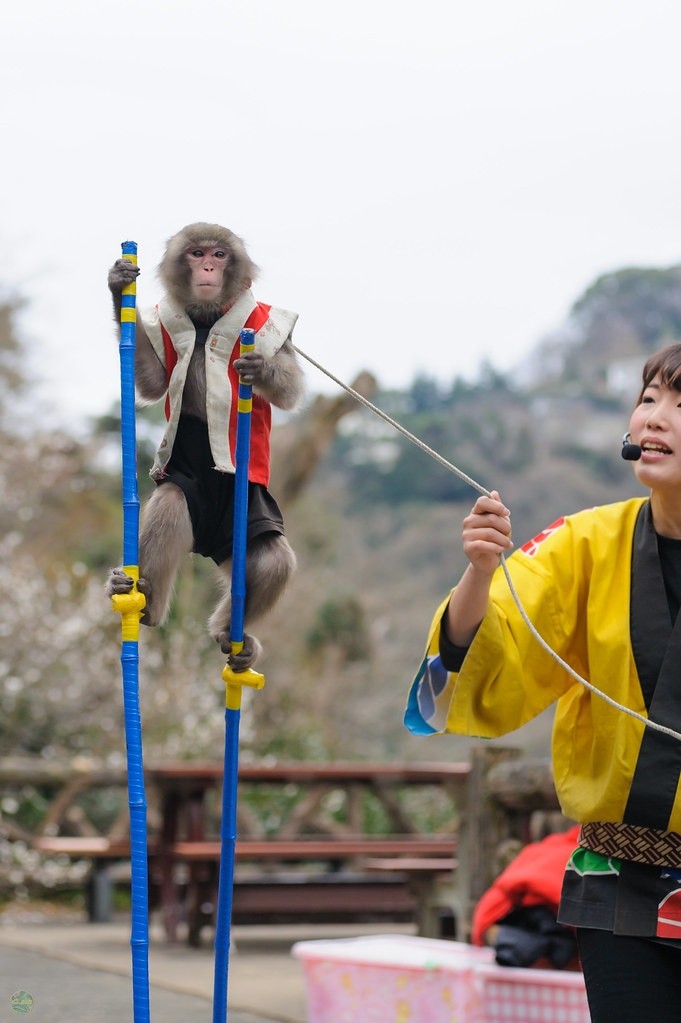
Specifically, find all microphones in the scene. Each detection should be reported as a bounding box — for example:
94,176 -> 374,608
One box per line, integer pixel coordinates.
621,432 -> 642,462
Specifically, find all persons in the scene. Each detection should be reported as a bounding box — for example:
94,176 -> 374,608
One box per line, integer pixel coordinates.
401,344 -> 681,1022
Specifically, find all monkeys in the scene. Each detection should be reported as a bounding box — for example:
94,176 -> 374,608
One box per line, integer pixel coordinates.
102,219 -> 304,676
